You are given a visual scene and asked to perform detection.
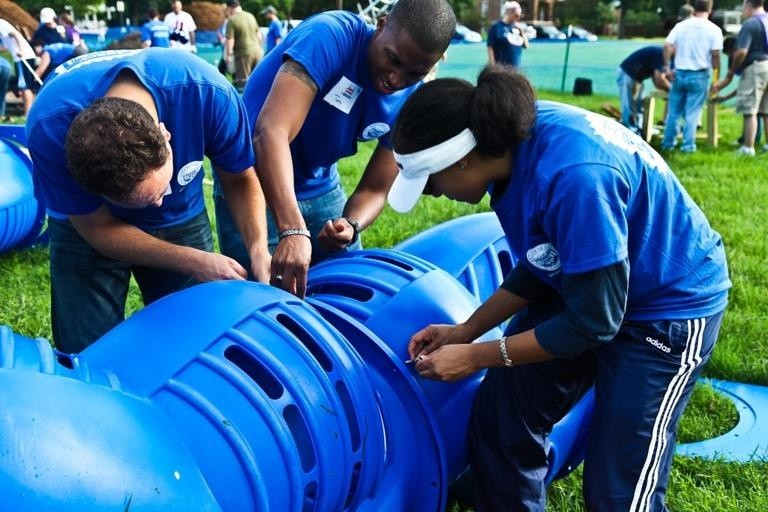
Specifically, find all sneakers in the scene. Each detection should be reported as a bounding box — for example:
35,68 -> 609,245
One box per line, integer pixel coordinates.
733,145 -> 756,158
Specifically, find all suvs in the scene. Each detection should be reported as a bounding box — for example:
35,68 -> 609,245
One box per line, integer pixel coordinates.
527,21 -> 567,40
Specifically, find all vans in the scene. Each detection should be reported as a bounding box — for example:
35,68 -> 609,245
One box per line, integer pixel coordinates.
710,10 -> 745,35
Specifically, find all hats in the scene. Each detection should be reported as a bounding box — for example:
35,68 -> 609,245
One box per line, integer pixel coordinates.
385,127 -> 477,214
39,7 -> 56,24
258,5 -> 277,16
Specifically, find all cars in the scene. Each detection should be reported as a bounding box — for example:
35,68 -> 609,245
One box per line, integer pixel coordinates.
515,19 -> 539,40
559,24 -> 598,42
454,25 -> 483,44
